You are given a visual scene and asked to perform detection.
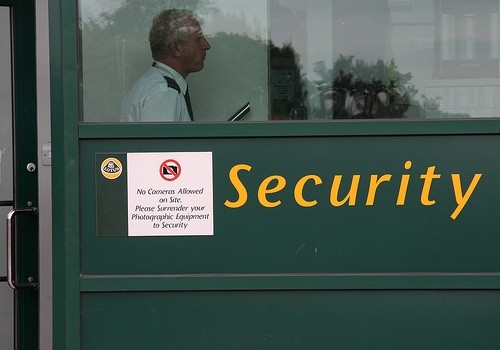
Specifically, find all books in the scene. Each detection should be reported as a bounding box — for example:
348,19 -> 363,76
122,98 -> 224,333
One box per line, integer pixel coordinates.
229,103 -> 251,121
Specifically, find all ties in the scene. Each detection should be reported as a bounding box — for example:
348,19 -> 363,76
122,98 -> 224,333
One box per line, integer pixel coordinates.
183,84 -> 194,121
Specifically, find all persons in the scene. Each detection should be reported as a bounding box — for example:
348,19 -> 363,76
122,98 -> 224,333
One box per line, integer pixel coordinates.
120,9 -> 211,122
